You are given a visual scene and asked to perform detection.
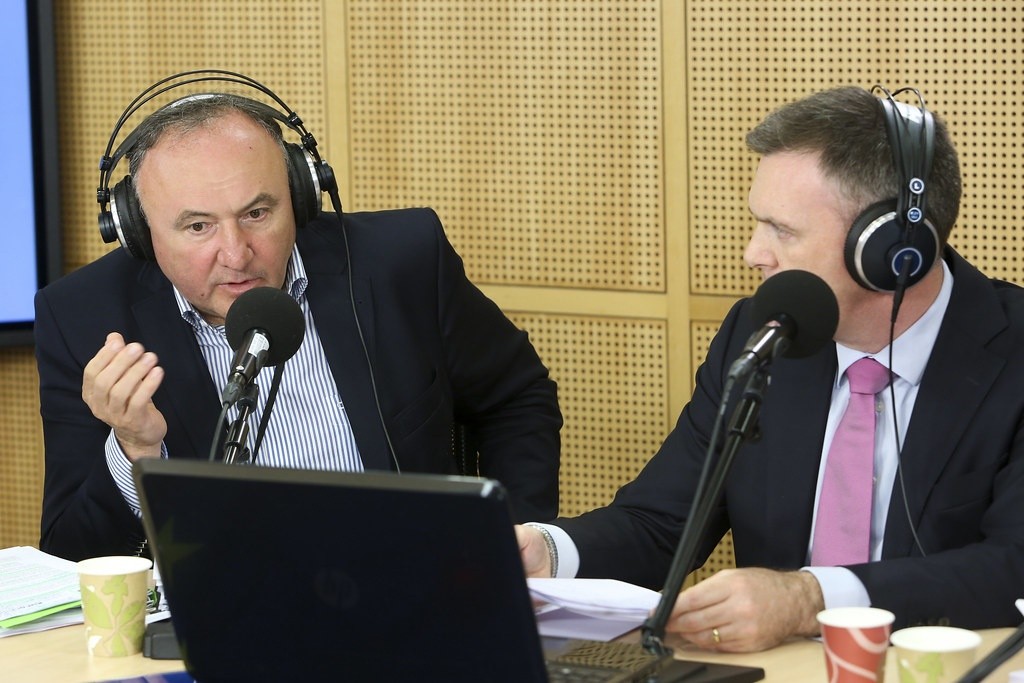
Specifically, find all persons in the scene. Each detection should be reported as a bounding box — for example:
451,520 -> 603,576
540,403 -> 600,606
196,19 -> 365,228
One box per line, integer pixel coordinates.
33,93 -> 564,563
514,86 -> 1023,654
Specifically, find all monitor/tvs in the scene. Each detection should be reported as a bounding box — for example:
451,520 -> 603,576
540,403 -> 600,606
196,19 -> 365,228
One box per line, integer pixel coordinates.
0,0 -> 65,348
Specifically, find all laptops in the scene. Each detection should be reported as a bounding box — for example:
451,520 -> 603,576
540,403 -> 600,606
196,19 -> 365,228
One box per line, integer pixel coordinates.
132,456 -> 767,683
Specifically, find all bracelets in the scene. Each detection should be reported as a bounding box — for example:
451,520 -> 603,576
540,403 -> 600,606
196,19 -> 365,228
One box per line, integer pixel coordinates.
526,525 -> 558,578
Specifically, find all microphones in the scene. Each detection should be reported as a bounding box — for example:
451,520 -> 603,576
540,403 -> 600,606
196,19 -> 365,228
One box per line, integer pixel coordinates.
222,286 -> 306,405
728,270 -> 840,380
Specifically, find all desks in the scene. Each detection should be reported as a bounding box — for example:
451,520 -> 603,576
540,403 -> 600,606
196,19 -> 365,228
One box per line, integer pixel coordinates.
0,623 -> 1024,683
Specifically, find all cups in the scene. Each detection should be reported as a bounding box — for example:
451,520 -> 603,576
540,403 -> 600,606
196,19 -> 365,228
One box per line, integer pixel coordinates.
890,626 -> 981,683
74,556 -> 153,660
815,607 -> 895,683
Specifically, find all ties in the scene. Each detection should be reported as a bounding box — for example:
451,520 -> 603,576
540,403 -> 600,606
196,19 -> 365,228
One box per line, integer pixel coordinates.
810,358 -> 894,567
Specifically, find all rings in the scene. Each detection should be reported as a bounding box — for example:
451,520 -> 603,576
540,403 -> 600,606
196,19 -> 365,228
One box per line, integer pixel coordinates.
713,629 -> 722,644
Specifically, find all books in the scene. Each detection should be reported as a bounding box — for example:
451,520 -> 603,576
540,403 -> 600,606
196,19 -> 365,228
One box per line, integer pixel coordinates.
0,545 -> 159,636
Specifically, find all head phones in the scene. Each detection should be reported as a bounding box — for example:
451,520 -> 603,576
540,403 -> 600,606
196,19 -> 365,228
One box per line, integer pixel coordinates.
843,85 -> 943,294
93,69 -> 341,265
